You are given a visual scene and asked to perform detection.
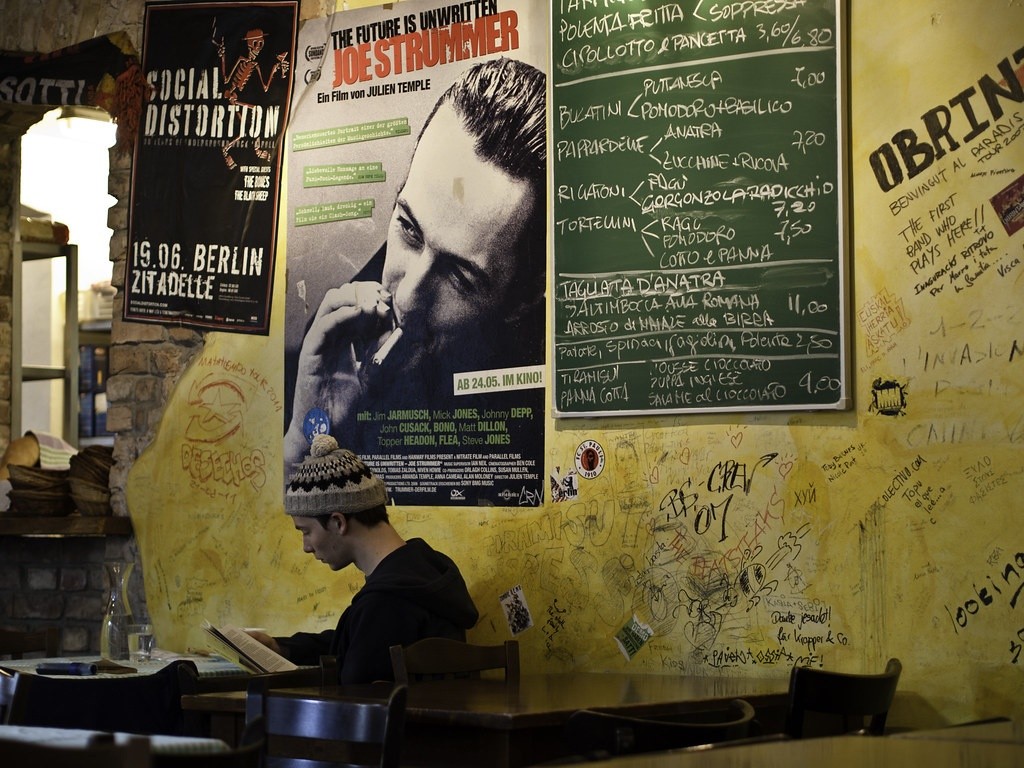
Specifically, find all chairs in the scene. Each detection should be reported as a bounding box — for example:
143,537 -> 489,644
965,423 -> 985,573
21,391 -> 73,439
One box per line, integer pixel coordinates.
391,640 -> 520,685
1,669 -> 41,726
240,683 -> 407,768
784,657 -> 903,740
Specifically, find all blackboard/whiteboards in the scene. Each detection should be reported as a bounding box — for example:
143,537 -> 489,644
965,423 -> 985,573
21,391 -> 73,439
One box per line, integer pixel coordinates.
542,1 -> 850,419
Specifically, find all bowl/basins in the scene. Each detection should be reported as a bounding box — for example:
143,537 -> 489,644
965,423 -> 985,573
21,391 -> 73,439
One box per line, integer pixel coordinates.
7,445 -> 116,517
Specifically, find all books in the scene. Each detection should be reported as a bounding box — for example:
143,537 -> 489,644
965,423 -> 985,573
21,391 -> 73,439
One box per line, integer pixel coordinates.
200,618 -> 300,675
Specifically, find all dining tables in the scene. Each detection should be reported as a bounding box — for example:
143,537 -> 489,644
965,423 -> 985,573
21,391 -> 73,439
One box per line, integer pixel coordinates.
180,672 -> 793,766
573,719 -> 1024,767
0,722 -> 238,767
0,651 -> 268,729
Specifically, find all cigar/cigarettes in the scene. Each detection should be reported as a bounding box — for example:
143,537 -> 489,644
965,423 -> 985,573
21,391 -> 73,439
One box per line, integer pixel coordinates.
369,326 -> 404,369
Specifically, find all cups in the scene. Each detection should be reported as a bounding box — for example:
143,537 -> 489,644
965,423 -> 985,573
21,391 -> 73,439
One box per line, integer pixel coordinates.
126,624 -> 154,662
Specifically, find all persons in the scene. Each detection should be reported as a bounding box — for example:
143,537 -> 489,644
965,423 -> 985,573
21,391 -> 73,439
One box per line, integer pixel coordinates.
285,58 -> 549,472
240,465 -> 480,683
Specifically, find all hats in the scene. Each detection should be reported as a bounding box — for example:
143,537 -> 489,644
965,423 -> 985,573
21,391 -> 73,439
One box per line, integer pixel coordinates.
283,433 -> 387,516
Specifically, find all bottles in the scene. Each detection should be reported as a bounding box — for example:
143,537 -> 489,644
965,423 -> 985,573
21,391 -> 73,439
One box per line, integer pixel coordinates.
100,561 -> 136,663
